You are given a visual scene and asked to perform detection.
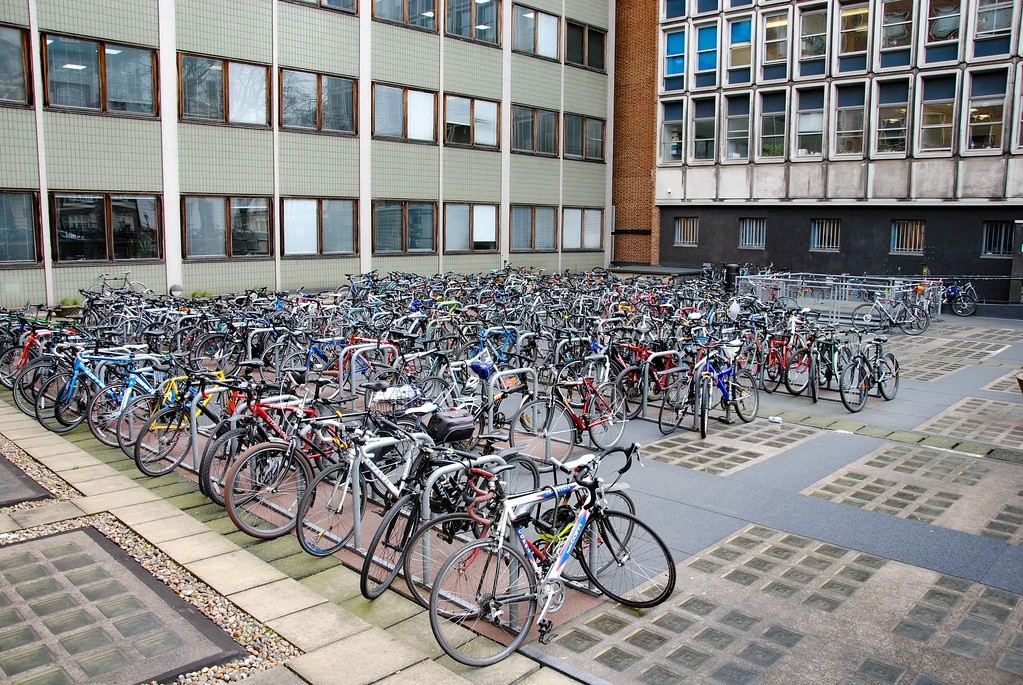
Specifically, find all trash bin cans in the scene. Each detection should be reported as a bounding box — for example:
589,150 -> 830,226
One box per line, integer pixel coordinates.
725,264 -> 740,291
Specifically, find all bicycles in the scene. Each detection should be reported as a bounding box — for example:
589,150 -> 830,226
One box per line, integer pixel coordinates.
0,269 -> 978,670
